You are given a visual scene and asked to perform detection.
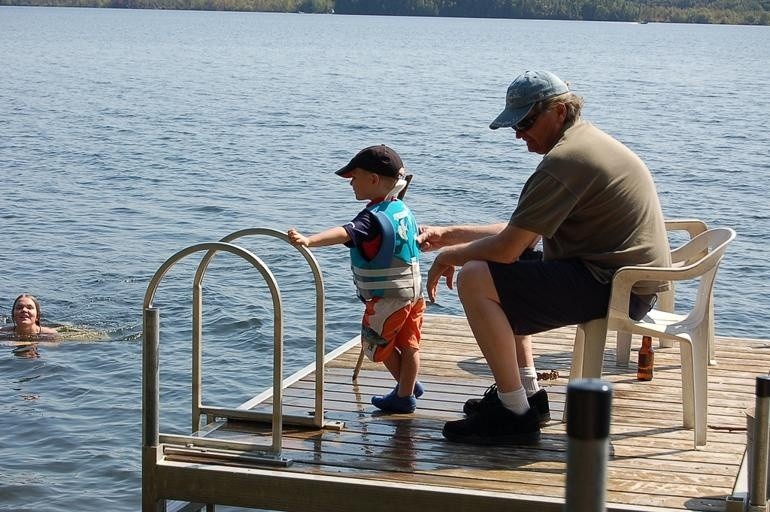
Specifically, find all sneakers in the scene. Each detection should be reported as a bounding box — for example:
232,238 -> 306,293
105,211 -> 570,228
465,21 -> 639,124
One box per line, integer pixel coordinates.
371,381 -> 424,413
442,383 -> 551,444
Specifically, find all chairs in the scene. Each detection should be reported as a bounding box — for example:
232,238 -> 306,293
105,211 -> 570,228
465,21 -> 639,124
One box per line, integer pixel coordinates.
571,218 -> 737,446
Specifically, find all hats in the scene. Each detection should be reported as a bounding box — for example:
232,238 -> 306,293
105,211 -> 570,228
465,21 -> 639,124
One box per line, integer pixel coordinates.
334,143 -> 405,179
488,69 -> 569,130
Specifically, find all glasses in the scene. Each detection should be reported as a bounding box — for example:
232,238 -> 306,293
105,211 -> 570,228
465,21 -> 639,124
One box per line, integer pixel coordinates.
512,106 -> 548,131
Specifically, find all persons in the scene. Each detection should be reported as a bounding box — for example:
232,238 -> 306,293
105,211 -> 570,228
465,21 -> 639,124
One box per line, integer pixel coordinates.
416,70 -> 670,445
287,145 -> 424,413
0,294 -> 111,346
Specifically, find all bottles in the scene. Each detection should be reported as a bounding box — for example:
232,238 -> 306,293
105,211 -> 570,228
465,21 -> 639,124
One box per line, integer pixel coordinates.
637,336 -> 654,381
